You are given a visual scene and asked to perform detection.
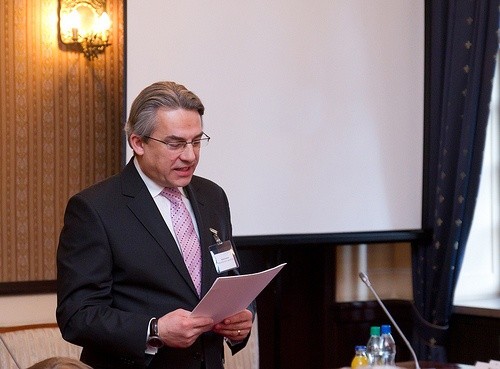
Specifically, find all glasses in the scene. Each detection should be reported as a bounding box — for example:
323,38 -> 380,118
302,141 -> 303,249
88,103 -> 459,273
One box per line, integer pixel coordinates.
139,131 -> 210,151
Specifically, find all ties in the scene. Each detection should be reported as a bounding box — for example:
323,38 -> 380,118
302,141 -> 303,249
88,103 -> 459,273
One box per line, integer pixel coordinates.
160,186 -> 203,299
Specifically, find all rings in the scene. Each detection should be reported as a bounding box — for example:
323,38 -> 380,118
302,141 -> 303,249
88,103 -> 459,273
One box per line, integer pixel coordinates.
238,329 -> 240,336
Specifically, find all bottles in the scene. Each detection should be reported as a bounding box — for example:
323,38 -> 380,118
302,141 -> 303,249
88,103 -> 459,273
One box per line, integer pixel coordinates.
379,325 -> 396,369
350,346 -> 368,369
366,326 -> 382,369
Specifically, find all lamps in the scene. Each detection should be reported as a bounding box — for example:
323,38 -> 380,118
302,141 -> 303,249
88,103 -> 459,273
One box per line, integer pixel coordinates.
57,0 -> 113,62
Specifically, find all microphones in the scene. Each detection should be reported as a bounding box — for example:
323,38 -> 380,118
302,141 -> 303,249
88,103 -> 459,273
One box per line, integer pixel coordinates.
359,272 -> 421,369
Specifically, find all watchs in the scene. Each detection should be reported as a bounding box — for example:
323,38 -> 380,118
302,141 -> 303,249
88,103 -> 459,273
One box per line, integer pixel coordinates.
147,319 -> 164,348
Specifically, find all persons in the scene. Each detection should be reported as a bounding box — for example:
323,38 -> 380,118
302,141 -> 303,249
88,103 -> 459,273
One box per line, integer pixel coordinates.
56,81 -> 256,369
